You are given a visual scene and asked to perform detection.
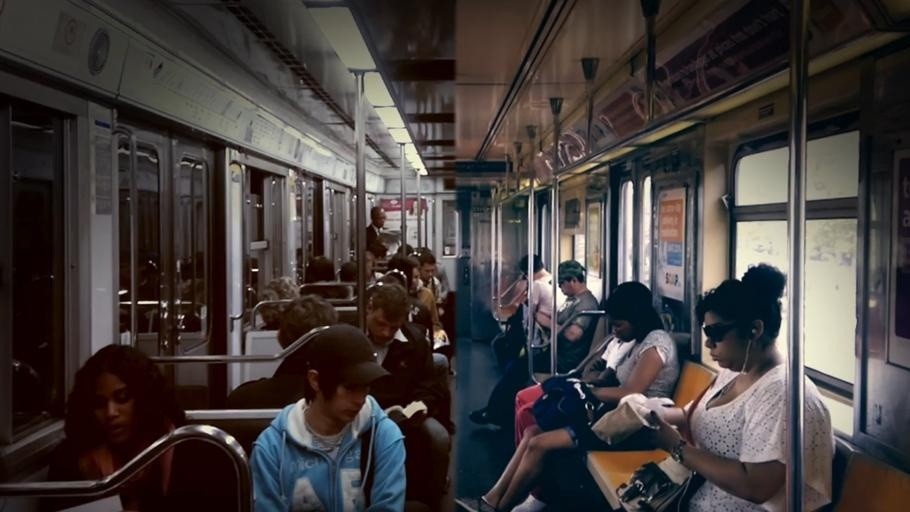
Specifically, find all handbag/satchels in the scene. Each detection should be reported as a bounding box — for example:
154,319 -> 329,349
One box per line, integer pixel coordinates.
615,456 -> 706,512
531,374 -> 607,434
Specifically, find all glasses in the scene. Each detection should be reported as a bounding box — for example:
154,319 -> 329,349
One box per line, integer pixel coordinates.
702,323 -> 740,343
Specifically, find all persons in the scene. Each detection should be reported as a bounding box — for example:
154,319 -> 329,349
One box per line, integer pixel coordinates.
451,276 -> 681,511
510,334 -> 638,511
246,321 -> 408,512
609,260 -> 835,512
39,344 -> 220,511
363,205 -> 390,256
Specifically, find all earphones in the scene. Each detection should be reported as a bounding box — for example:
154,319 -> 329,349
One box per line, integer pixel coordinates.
753,329 -> 756,334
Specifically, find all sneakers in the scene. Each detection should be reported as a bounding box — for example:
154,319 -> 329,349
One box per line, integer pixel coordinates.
469,407 -> 491,426
454,494 -> 496,512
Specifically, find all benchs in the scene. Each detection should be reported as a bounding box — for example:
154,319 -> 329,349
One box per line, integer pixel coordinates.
0,251 -> 441,512
490,267 -> 910,512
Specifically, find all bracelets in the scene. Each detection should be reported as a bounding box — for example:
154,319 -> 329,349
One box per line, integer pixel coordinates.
667,436 -> 687,465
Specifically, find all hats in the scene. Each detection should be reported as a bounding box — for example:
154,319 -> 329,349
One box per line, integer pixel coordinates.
548,260 -> 587,285
303,324 -> 392,387
